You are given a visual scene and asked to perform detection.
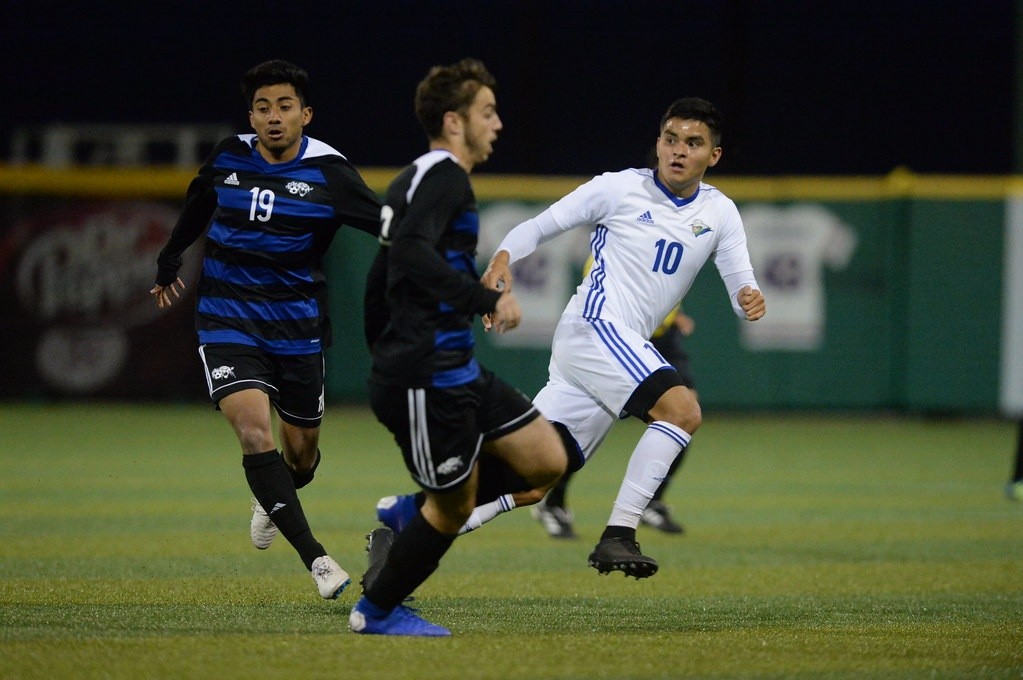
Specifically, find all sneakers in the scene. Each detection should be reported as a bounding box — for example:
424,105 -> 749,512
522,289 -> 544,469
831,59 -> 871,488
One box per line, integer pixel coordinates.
250,497 -> 279,550
587,537 -> 659,580
530,495 -> 573,537
310,555 -> 351,599
374,493 -> 416,534
359,528 -> 393,596
641,500 -> 683,533
347,595 -> 453,637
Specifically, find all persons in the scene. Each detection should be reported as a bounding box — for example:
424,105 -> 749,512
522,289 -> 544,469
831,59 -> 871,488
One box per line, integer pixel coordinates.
530,254 -> 697,535
346,62 -> 568,635
359,99 -> 766,597
151,63 -> 382,598
